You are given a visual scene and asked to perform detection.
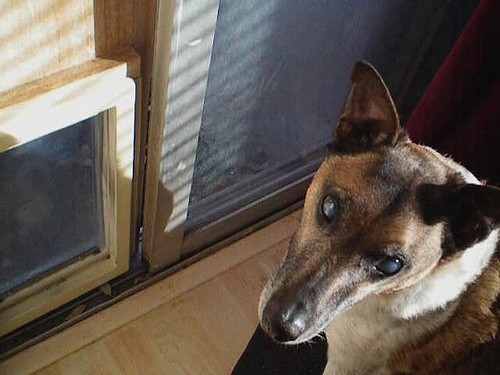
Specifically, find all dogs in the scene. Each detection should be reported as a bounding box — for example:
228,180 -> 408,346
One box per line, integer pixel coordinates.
257,59 -> 500,375
